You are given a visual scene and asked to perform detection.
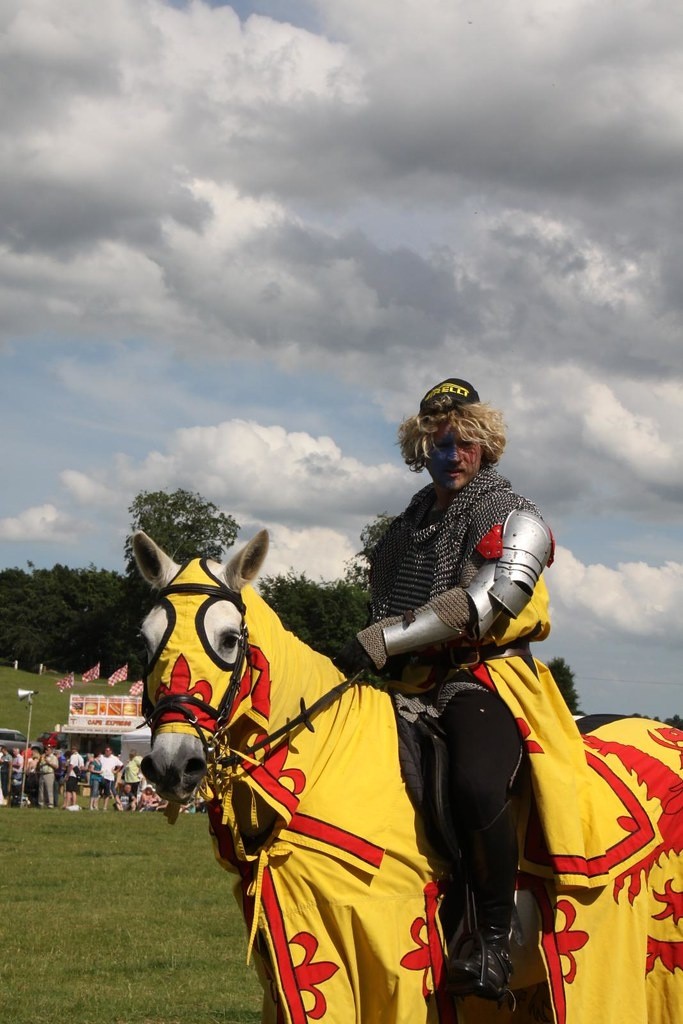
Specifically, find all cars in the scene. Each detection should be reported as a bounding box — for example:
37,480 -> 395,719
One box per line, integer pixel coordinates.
0,728 -> 44,756
36,731 -> 67,748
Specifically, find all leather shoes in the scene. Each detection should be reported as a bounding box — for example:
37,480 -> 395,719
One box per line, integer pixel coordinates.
446,936 -> 515,1000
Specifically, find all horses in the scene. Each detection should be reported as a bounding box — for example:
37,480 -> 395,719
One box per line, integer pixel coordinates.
129,529 -> 682,1024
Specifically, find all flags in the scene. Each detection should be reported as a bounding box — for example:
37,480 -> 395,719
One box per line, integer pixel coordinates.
82,662 -> 100,684
108,665 -> 127,687
129,681 -> 144,696
55,672 -> 74,693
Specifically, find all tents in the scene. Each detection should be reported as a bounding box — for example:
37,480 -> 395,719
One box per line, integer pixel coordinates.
121,726 -> 152,764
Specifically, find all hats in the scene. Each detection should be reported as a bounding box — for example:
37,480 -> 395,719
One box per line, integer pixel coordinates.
45,744 -> 53,750
419,378 -> 480,417
72,746 -> 79,750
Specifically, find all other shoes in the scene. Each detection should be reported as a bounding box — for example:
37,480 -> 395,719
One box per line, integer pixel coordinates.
89,806 -> 93,811
39,802 -> 43,806
48,805 -> 54,808
95,807 -> 100,811
60,805 -> 67,809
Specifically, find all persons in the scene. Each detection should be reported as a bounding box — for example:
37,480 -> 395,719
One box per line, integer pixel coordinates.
0,739 -> 213,816
332,378 -> 556,1000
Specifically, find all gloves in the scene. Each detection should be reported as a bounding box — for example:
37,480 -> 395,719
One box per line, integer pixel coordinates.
333,638 -> 372,679
